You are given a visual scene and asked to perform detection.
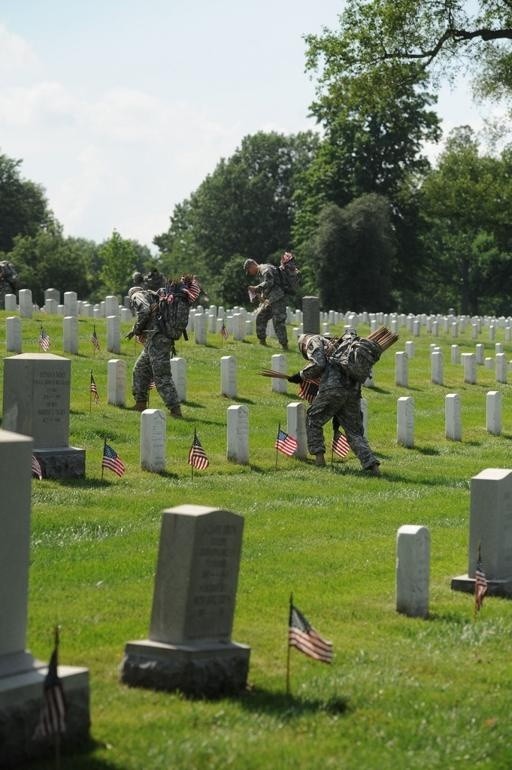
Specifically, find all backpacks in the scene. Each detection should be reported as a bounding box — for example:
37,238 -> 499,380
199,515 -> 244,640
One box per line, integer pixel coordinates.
149,288 -> 189,340
328,334 -> 380,384
275,257 -> 298,294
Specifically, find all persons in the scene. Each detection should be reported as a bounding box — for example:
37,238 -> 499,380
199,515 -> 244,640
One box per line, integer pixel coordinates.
243,258 -> 289,350
298,334 -> 381,469
0,260 -> 17,310
126,267 -> 181,418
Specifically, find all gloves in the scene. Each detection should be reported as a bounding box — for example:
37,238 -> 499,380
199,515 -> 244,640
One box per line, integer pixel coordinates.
289,374 -> 301,383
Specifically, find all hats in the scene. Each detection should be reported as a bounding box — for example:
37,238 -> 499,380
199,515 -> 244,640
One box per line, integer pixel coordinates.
243,259 -> 253,276
298,334 -> 307,359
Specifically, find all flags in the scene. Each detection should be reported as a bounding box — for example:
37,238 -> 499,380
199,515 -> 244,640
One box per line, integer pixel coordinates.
289,606 -> 334,665
475,552 -> 488,610
183,276 -> 202,304
33,634 -> 67,740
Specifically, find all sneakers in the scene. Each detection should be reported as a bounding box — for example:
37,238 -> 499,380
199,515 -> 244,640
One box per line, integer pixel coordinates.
171,406 -> 181,417
371,465 -> 379,476
315,454 -> 325,466
134,401 -> 145,410
